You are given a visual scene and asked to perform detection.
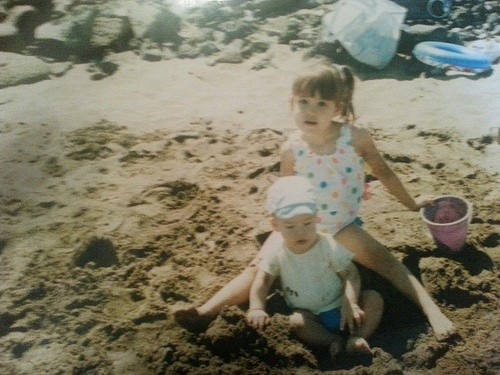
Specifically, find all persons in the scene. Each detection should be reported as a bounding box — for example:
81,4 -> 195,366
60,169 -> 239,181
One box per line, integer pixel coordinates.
248,176 -> 384,358
173,66 -> 457,341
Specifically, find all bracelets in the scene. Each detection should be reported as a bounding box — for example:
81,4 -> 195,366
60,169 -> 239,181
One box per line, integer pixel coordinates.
248,306 -> 264,314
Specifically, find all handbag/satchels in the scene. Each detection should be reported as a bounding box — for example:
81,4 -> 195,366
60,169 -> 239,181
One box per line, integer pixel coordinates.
324,0 -> 408,70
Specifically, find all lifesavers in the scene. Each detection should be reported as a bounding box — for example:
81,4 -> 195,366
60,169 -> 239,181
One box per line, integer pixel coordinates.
412,41 -> 493,73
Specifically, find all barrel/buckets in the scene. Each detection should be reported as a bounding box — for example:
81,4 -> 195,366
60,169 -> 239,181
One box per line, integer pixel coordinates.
420,195 -> 471,253
